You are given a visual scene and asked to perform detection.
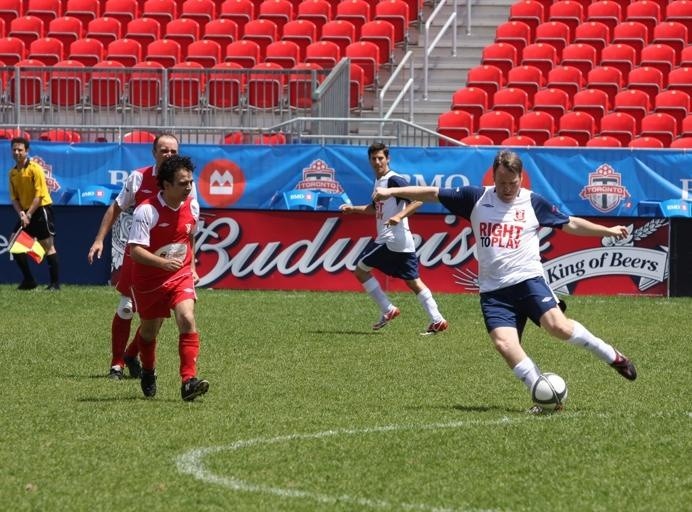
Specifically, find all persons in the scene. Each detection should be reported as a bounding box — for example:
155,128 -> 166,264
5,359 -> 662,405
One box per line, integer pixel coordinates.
86,132 -> 197,378
339,142 -> 449,335
369,148 -> 638,413
129,156 -> 209,401
6,137 -> 63,293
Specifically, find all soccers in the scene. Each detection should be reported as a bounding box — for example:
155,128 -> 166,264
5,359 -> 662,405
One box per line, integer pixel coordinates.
530,373 -> 568,410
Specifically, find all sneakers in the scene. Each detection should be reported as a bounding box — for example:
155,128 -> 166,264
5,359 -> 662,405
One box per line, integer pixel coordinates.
372,305 -> 400,331
141,367 -> 156,397
610,348 -> 637,381
110,352 -> 141,379
18,279 -> 59,290
427,320 -> 448,333
181,377 -> 210,401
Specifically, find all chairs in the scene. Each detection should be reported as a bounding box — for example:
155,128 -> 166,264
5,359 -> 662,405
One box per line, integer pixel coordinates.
437,0 -> 692,149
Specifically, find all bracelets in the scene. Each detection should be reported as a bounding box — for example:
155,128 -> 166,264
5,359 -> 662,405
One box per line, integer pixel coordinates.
26,212 -> 33,220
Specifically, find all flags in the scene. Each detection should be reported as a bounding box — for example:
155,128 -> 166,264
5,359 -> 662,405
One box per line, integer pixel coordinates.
9,224 -> 47,267
